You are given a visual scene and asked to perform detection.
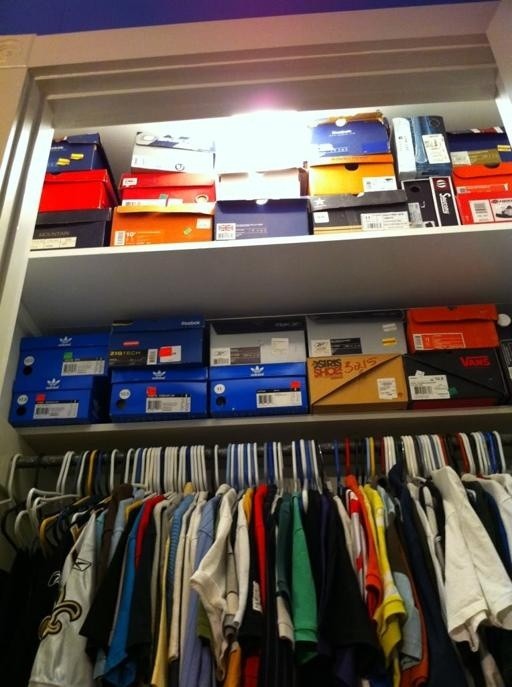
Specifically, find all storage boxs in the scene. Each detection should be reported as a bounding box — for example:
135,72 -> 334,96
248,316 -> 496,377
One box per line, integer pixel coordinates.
306,111 -> 394,155
402,302 -> 499,352
305,354 -> 407,414
306,152 -> 397,196
107,322 -> 209,366
312,185 -> 412,236
309,309 -> 407,358
399,176 -> 462,225
498,304 -> 512,409
31,209 -> 109,251
109,206 -> 214,245
210,360 -> 311,417
8,373 -> 105,425
129,132 -> 216,176
11,327 -> 113,375
447,124 -> 512,166
215,160 -> 305,202
39,171 -> 118,209
102,368 -> 211,422
119,169 -> 216,203
213,194 -> 312,244
203,314 -> 310,368
450,161 -> 512,224
46,133 -> 117,192
392,113 -> 453,180
406,350 -> 512,414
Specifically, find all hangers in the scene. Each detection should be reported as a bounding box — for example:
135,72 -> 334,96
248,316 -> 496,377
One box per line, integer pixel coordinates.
2,427 -> 508,552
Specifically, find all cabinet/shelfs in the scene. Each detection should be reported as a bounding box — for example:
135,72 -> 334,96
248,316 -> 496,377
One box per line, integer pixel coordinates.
0,31 -> 512,687
